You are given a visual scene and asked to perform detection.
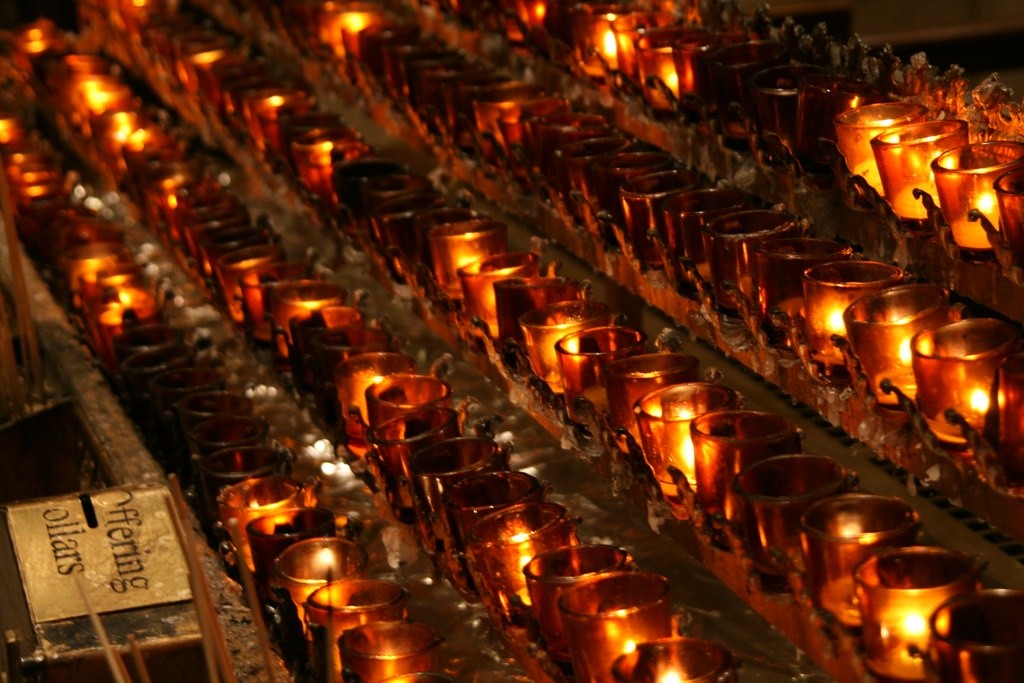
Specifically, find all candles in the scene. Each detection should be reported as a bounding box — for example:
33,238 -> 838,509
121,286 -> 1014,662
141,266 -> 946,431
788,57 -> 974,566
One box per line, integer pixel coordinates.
0,0 -> 1024,683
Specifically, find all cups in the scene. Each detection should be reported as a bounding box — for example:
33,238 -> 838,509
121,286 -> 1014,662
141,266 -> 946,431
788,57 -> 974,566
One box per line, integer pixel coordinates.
0,0 -> 1024,683
